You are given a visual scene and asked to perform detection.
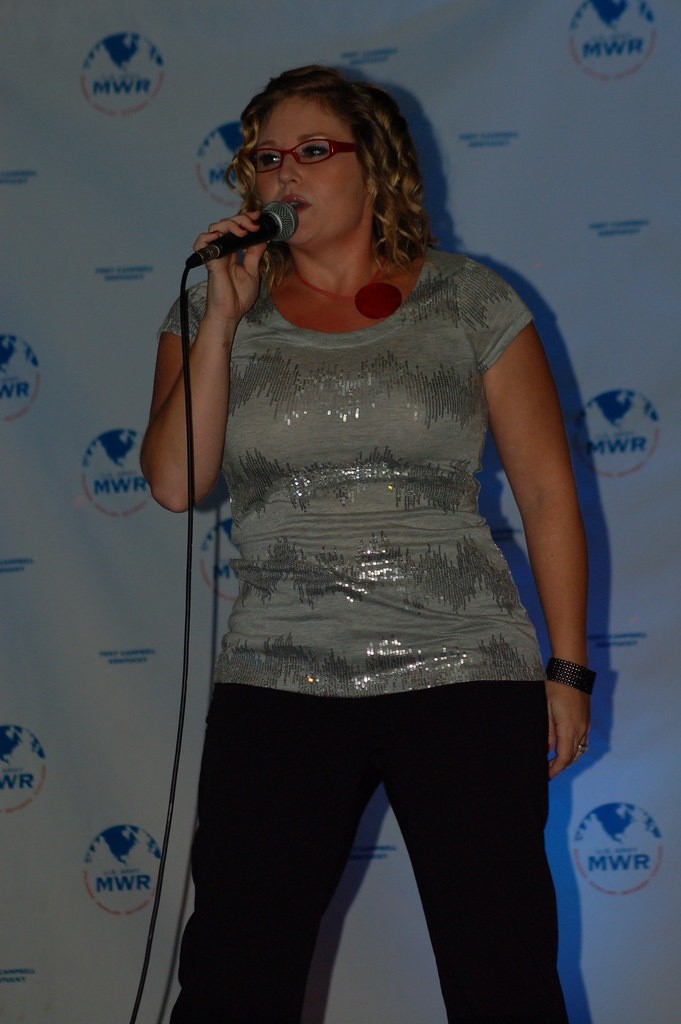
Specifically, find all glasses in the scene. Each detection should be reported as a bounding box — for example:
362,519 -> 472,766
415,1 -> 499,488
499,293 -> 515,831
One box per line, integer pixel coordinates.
251,139 -> 360,172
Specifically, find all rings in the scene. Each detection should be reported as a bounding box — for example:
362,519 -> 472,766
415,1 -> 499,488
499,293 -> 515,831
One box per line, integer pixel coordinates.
579,744 -> 589,753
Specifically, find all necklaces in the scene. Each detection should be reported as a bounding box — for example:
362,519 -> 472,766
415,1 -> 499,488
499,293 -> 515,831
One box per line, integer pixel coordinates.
293,258 -> 402,318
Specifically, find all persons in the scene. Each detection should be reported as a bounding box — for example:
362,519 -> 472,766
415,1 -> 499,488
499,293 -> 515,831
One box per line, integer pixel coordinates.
140,66 -> 597,1024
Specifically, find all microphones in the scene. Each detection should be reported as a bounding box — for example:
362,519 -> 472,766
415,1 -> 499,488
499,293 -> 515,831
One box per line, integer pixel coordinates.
185,200 -> 299,269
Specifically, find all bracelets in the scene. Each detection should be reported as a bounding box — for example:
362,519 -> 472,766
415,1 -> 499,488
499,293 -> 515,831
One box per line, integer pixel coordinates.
546,658 -> 596,695
